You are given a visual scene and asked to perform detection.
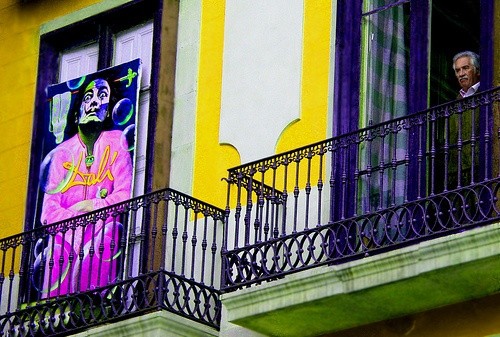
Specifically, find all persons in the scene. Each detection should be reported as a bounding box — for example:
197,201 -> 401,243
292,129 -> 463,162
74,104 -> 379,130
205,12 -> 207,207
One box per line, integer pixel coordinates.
436,51 -> 489,224
